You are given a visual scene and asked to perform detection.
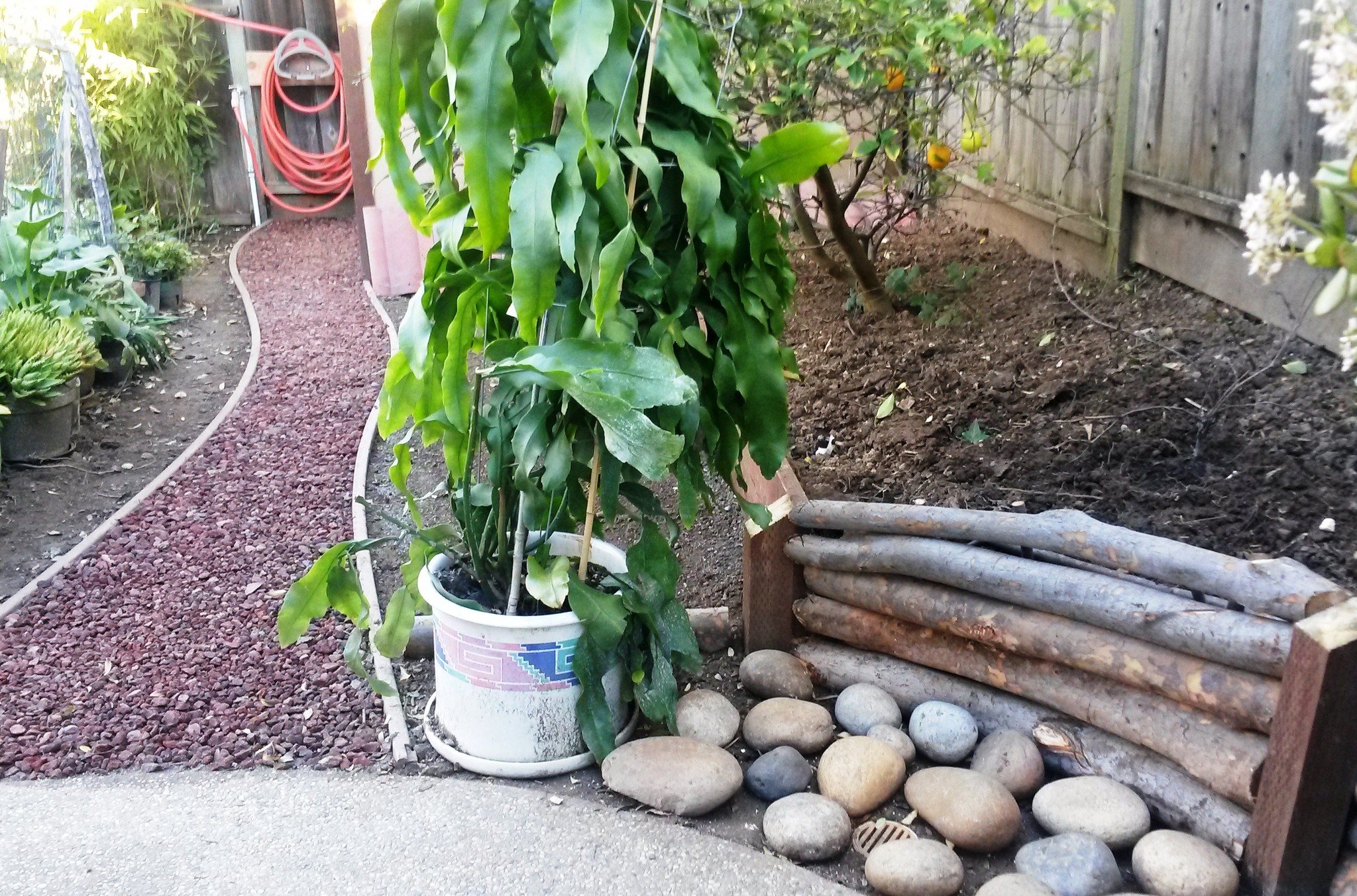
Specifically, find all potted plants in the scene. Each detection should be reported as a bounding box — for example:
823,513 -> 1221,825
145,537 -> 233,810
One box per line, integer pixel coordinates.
273,0 -> 848,785
1,184 -> 197,462
